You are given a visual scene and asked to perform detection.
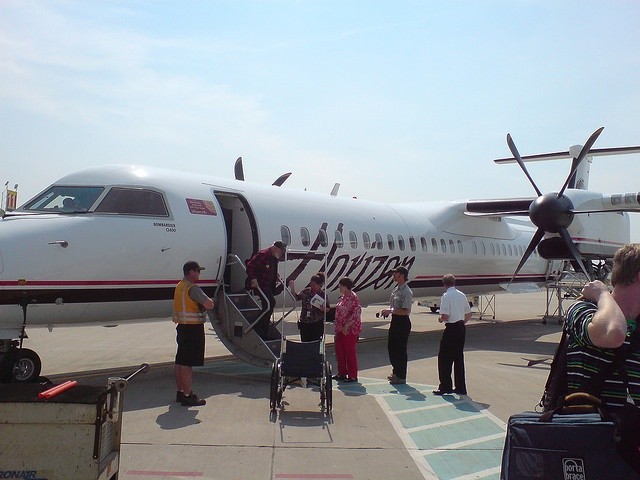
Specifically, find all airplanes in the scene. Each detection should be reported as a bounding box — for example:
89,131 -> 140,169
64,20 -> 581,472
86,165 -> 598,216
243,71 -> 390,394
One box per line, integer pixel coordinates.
1,126 -> 640,386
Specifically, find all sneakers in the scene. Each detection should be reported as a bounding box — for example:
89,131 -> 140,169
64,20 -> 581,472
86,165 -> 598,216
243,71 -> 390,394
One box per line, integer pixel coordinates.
388,376 -> 398,380
182,391 -> 206,406
390,378 -> 406,384
177,391 -> 184,402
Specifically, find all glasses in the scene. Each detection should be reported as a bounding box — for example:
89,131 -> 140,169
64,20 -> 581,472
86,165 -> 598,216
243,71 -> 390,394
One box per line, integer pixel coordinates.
194,269 -> 200,273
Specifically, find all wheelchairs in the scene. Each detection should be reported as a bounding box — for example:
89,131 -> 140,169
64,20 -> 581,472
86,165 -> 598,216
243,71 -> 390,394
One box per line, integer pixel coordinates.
269,334 -> 331,414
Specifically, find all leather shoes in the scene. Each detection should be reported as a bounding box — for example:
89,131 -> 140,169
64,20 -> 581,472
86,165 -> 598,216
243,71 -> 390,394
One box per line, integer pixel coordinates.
433,390 -> 452,395
453,388 -> 467,394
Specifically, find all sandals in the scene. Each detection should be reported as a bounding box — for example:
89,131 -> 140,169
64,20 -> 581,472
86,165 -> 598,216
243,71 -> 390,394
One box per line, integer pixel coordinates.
332,374 -> 347,380
343,378 -> 357,382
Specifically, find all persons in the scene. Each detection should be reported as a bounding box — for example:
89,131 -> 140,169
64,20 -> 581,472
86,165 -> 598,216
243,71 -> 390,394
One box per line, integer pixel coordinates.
246,241 -> 286,340
332,278 -> 362,381
289,272 -> 331,387
380,267 -> 413,383
433,274 -> 473,395
542,243 -> 640,414
172,261 -> 214,407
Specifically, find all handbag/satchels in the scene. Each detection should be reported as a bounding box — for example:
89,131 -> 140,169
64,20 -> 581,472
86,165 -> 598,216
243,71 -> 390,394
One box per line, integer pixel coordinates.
500,392 -> 640,480
272,278 -> 284,295
535,335 -> 567,413
602,402 -> 640,476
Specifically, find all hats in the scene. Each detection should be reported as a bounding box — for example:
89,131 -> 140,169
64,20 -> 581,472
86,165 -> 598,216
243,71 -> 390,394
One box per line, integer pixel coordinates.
390,267 -> 408,275
274,241 -> 286,252
184,261 -> 205,274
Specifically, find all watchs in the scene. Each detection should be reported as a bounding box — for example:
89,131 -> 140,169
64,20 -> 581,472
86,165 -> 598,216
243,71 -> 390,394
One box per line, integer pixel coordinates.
391,307 -> 395,314
